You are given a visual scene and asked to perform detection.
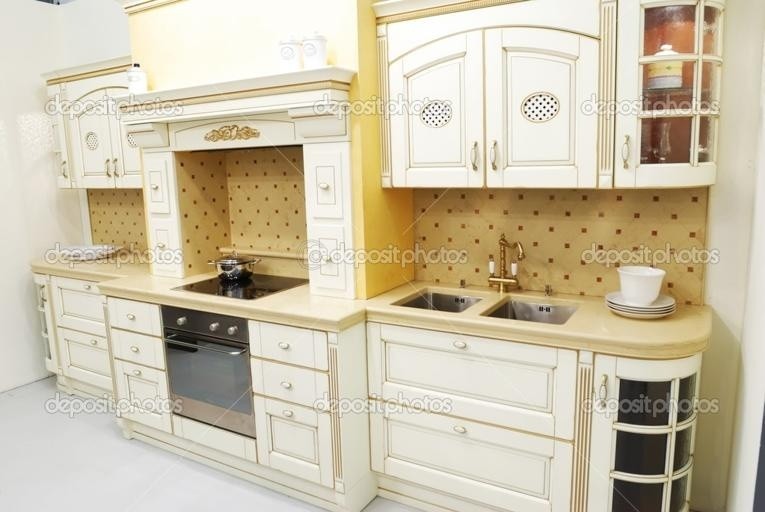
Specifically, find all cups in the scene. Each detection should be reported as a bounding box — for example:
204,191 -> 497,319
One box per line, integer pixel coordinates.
653,122 -> 673,163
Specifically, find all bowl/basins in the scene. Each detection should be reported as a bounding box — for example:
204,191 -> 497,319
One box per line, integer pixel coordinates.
617,265 -> 666,305
648,45 -> 684,89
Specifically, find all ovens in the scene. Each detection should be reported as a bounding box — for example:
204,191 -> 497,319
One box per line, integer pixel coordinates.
162,329 -> 256,440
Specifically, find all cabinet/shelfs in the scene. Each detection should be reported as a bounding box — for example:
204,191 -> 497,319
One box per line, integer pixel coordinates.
30,272 -> 59,374
365,321 -> 578,512
103,296 -> 173,435
387,0 -> 599,189
248,319 -> 371,494
46,70 -> 141,191
49,273 -> 113,393
613,1 -> 726,190
587,352 -> 702,512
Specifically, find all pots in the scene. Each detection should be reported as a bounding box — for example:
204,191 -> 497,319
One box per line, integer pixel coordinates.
209,250 -> 259,280
214,281 -> 263,300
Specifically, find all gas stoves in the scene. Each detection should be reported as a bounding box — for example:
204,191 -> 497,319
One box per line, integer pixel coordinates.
160,272 -> 310,343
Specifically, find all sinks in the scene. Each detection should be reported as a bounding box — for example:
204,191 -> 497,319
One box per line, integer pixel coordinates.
390,286 -> 491,313
478,294 -> 581,326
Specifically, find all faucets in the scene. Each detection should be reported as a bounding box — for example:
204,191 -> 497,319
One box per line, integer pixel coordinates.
498,231 -> 525,280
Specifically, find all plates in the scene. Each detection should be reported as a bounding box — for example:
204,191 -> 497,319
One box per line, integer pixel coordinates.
64,243 -> 124,260
605,290 -> 679,320
